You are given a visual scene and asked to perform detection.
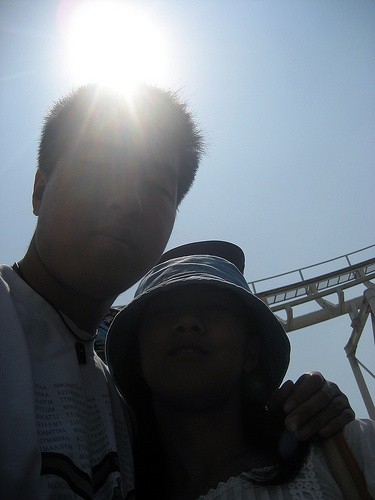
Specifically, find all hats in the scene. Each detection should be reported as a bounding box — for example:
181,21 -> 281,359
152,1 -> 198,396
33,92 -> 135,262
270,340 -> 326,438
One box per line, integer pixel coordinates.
104,254 -> 290,413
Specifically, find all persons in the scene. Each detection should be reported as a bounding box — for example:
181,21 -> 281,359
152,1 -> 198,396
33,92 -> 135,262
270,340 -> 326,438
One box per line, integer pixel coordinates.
1,83 -> 358,500
105,254 -> 375,500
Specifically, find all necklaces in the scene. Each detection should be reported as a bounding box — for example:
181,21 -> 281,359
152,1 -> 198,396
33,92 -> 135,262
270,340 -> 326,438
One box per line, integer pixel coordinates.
13,262 -> 99,364
